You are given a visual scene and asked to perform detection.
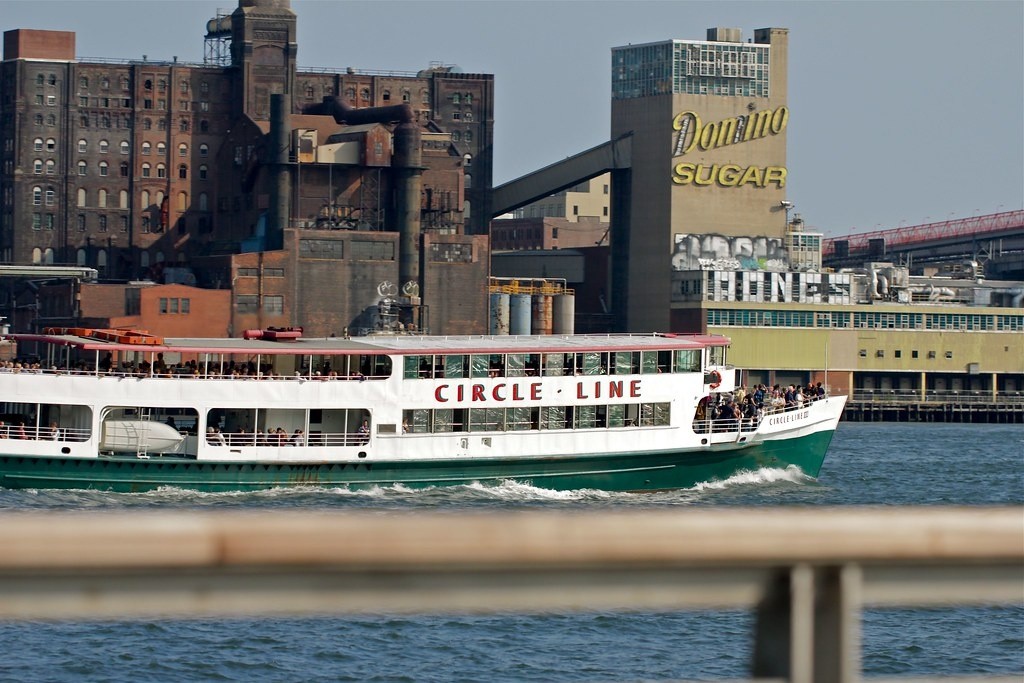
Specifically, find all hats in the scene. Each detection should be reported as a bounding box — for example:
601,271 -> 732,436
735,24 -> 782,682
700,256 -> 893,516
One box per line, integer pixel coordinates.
208,427 -> 214,432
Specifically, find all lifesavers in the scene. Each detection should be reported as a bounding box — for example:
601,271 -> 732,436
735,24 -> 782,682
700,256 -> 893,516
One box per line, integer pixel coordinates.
709,369 -> 720,388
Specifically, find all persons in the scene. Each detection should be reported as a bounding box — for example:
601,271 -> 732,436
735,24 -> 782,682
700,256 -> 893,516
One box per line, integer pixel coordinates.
0,353 -> 826,446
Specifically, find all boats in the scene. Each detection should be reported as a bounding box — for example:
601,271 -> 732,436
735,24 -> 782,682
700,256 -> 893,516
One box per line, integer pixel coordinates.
0,318 -> 849,492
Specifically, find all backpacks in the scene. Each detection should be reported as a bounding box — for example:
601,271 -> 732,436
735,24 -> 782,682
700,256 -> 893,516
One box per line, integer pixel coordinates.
694,406 -> 701,419
